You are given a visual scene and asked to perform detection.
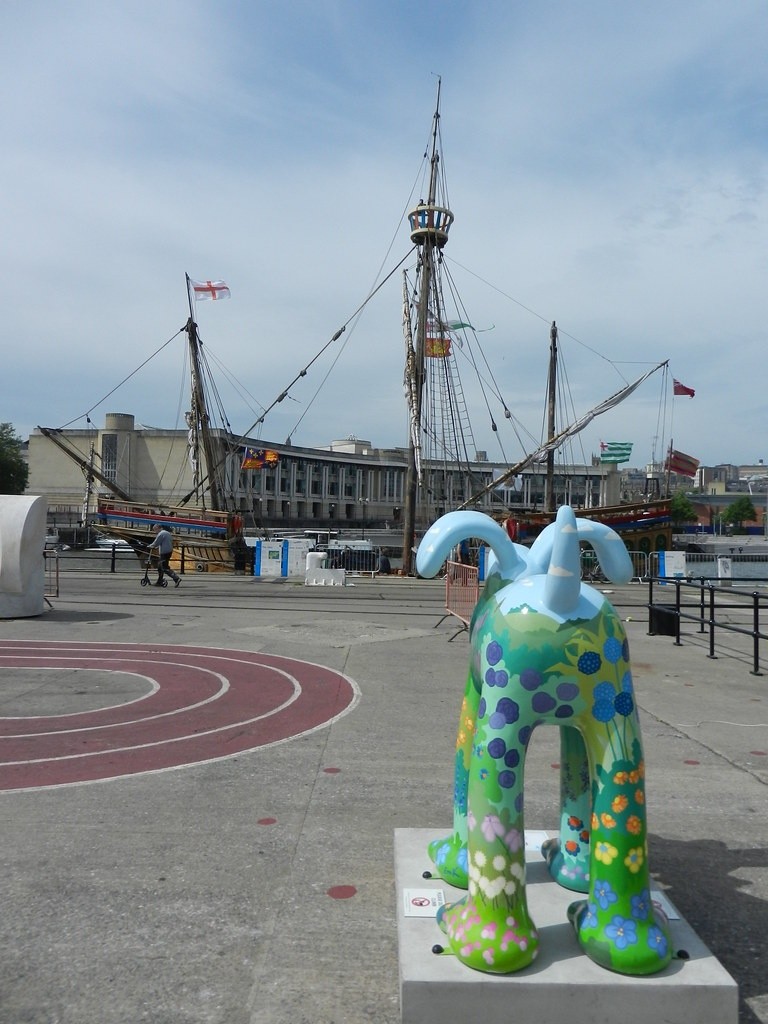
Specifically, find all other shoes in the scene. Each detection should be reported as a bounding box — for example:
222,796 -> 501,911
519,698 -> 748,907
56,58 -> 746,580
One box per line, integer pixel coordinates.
175,578 -> 181,588
151,583 -> 160,586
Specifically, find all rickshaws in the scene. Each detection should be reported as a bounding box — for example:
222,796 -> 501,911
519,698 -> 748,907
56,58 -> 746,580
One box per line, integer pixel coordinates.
141,546 -> 168,587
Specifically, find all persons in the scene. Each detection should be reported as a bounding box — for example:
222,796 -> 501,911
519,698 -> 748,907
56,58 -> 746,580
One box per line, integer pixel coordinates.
148,524 -> 182,588
376,548 -> 391,575
231,533 -> 248,575
449,538 -> 472,587
331,550 -> 350,570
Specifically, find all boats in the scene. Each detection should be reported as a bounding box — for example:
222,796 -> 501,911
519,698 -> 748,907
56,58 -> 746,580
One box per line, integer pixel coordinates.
38,71 -> 687,576
244,530 -> 374,563
95,540 -> 132,551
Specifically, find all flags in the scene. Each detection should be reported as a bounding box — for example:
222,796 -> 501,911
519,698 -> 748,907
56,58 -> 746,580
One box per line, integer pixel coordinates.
601,442 -> 633,463
664,447 -> 700,477
425,338 -> 452,358
411,299 -> 495,332
189,278 -> 231,301
673,378 -> 695,399
241,446 -> 278,470
